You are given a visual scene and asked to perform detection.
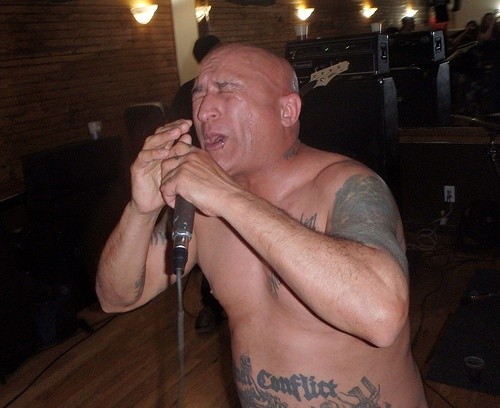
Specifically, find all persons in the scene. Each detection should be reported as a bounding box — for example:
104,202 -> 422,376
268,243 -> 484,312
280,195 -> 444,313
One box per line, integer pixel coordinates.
169,35 -> 220,148
399,17 -> 414,33
96,41 -> 428,408
444,13 -> 500,116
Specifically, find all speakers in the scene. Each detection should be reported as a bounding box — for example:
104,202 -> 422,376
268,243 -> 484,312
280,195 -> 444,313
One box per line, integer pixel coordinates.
389,62 -> 450,128
294,76 -> 400,196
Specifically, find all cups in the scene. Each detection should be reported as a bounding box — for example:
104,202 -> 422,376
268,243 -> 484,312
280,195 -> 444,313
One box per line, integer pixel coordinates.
88,121 -> 102,141
371,23 -> 382,34
295,24 -> 309,41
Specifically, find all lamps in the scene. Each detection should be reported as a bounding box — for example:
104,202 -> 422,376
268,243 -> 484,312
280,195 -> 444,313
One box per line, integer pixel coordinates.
295,7 -> 315,21
360,7 -> 378,19
130,3 -> 158,25
402,9 -> 419,18
195,5 -> 212,23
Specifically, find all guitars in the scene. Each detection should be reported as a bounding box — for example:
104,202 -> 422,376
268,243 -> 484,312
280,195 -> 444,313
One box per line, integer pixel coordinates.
298,61 -> 350,97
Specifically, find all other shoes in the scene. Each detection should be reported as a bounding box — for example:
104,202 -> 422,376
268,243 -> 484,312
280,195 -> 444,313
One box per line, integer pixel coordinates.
197,306 -> 223,332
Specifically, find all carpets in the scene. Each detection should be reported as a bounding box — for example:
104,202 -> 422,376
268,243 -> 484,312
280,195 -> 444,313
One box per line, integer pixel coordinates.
419,268 -> 500,397
